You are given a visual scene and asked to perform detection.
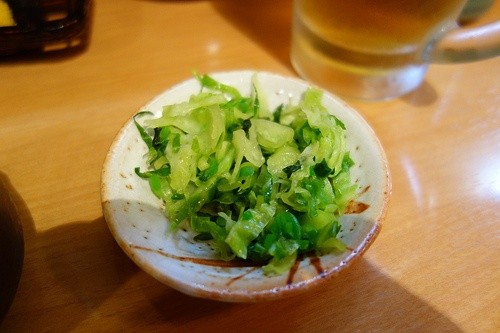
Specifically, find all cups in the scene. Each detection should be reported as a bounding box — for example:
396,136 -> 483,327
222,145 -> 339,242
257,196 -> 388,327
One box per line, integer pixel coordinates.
290,0 -> 469,102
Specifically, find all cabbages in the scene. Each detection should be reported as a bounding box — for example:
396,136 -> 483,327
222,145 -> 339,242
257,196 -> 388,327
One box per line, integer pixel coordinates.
131,70 -> 358,274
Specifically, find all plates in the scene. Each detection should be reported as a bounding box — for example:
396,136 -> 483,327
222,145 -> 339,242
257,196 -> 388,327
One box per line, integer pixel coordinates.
100,70 -> 393,303
